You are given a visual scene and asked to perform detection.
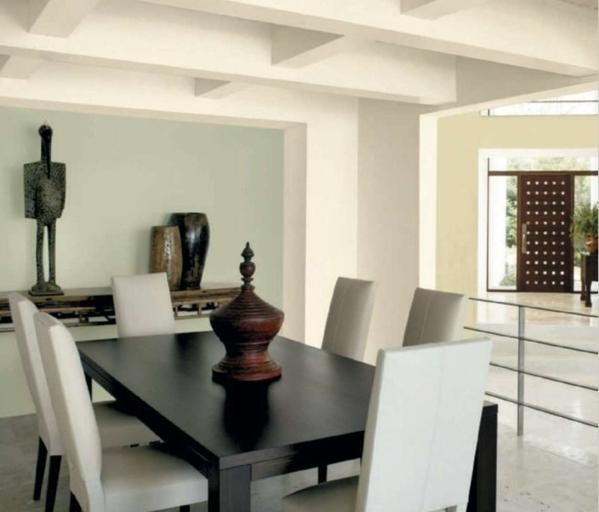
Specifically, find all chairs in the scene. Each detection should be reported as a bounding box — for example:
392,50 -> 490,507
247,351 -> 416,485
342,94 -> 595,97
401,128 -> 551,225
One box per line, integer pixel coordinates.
34,311 -> 215,512
321,276 -> 378,360
111,272 -> 174,337
282,336 -> 492,512
9,292 -> 163,512
319,288 -> 467,483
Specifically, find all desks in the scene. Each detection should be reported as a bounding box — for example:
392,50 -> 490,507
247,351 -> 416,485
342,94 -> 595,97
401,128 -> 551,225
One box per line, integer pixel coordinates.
580,252 -> 598,307
0,284 -> 254,334
76,330 -> 498,512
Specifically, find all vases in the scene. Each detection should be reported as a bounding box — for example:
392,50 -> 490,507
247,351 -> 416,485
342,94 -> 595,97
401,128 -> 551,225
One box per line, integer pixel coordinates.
169,213 -> 209,290
149,225 -> 182,290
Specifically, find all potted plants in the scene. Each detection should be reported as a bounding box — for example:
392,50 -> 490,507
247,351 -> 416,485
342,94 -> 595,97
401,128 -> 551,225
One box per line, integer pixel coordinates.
571,202 -> 598,253
